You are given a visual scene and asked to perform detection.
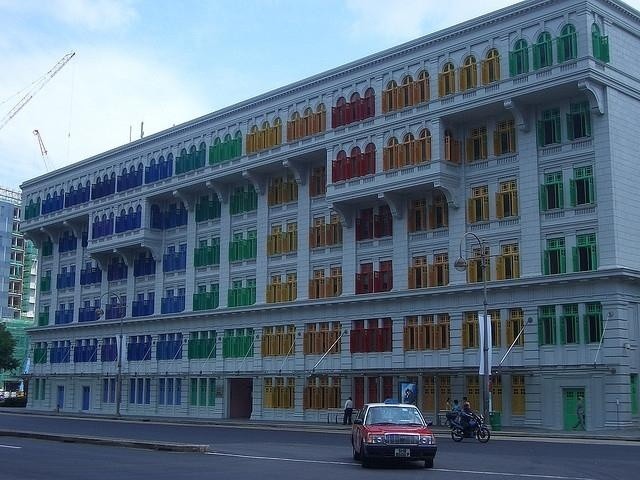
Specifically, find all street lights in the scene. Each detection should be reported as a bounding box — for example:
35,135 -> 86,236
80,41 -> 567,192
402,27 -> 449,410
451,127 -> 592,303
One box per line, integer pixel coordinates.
454,230 -> 492,431
95,291 -> 123,418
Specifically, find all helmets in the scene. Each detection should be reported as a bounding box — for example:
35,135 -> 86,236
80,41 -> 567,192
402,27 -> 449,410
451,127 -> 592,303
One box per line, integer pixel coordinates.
463,402 -> 471,410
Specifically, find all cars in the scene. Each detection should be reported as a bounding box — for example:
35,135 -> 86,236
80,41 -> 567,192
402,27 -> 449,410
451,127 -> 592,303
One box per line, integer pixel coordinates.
351,402 -> 437,469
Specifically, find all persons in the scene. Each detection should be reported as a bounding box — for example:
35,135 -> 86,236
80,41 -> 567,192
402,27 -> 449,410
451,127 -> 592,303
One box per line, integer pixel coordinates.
459,404 -> 475,435
341,396 -> 354,426
463,396 -> 471,410
453,400 -> 460,412
443,398 -> 453,425
572,396 -> 587,432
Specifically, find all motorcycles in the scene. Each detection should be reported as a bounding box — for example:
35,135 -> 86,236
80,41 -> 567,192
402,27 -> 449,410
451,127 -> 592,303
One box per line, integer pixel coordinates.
447,410 -> 491,443
